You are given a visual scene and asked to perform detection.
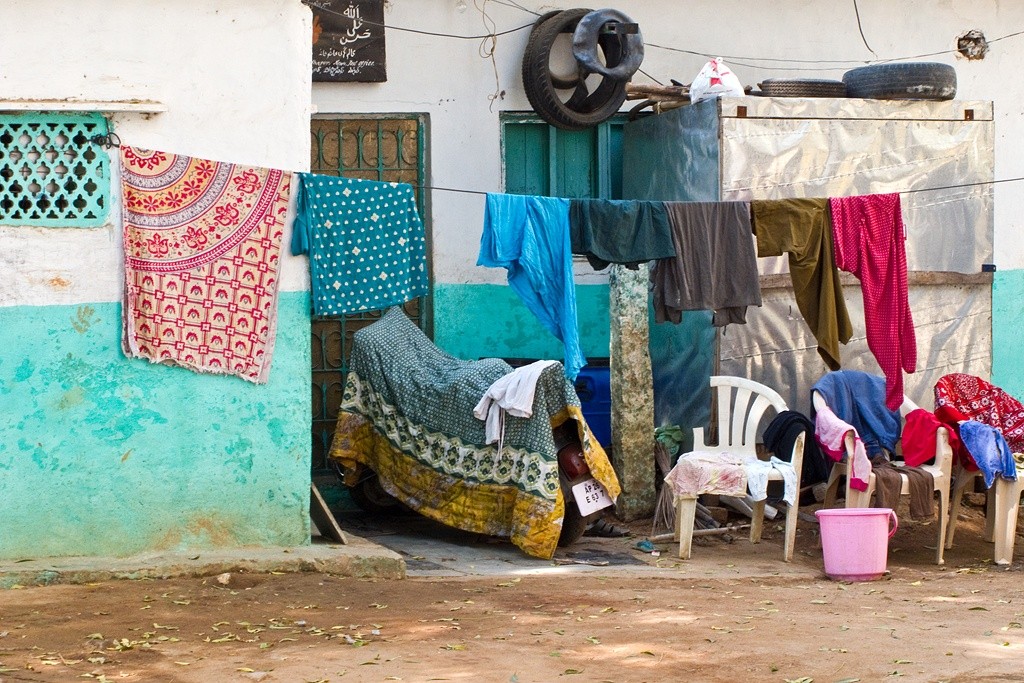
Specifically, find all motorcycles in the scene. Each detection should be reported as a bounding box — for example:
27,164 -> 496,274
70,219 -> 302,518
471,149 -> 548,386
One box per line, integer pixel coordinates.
352,309 -> 615,546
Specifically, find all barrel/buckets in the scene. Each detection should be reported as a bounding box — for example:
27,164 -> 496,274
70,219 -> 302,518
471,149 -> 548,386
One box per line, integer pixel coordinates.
814,508 -> 899,582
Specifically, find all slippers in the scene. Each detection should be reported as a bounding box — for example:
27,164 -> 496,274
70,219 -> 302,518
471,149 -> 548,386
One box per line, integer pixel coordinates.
583,519 -> 630,536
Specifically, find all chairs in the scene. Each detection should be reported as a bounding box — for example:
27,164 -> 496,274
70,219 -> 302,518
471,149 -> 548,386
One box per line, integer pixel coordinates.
934,373 -> 1024,565
813,371 -> 952,564
674,376 -> 807,562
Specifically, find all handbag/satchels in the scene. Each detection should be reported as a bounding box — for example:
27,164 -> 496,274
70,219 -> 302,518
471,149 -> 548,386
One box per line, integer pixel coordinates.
689,57 -> 746,105
